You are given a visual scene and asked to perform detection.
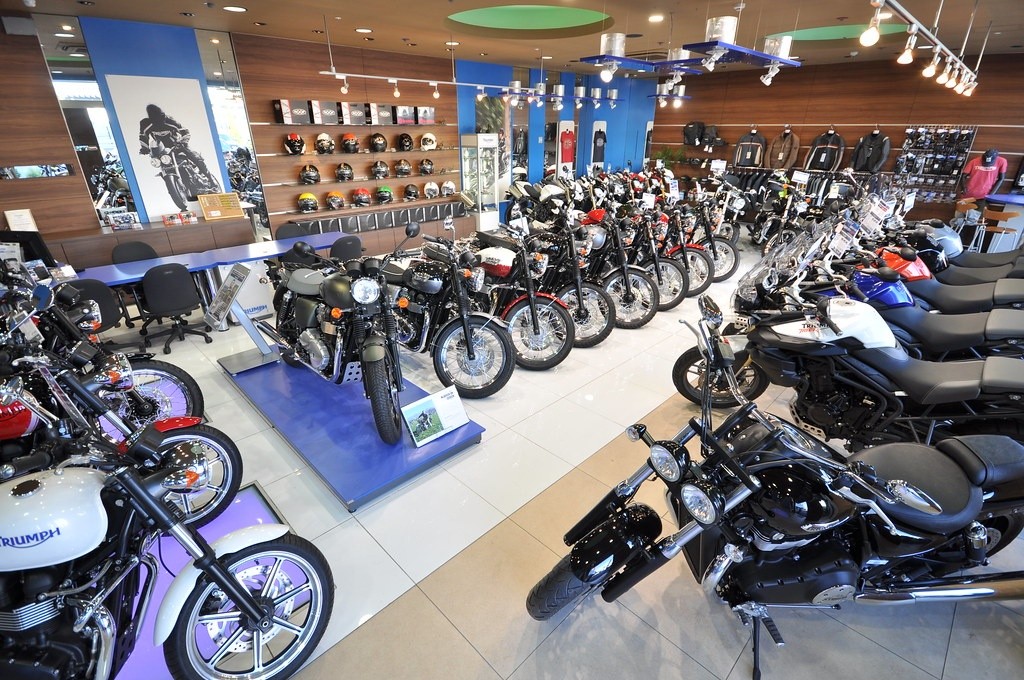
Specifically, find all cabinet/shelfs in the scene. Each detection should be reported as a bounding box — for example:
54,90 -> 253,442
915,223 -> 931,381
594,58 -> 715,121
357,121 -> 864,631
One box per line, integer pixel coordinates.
248,122 -> 461,216
459,133 -> 499,233
41,215 -> 255,270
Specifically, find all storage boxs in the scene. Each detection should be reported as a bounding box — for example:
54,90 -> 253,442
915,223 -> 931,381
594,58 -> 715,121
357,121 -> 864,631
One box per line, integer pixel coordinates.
96,206 -> 144,233
273,98 -> 435,125
163,213 -> 198,227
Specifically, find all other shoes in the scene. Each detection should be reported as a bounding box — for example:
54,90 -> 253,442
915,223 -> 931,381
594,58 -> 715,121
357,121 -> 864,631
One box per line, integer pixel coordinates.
205,171 -> 218,188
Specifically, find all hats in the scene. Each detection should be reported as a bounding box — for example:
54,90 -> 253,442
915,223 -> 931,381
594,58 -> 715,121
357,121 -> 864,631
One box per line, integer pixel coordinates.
982,149 -> 999,166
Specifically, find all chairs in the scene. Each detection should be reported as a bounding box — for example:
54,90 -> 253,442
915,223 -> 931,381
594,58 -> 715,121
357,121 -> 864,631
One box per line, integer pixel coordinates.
950,197 -> 1018,253
54,241 -> 214,354
263,223 -> 365,289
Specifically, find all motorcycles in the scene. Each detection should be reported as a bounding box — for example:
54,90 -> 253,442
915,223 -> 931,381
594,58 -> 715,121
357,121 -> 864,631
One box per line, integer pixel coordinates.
224,147 -> 270,228
673,168 -> 1024,452
138,135 -> 222,210
526,295 -> 1024,680
416,418 -> 432,435
260,160 -> 740,446
91,152 -> 137,212
0,259 -> 336,680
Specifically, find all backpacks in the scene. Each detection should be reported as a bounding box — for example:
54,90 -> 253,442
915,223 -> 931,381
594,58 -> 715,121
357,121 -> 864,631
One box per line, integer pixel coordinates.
701,125 -> 718,145
683,121 -> 705,145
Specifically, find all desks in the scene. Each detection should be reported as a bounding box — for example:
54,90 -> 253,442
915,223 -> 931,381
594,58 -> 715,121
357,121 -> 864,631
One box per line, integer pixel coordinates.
44,252 -> 229,333
202,231 -> 351,265
986,193 -> 1024,253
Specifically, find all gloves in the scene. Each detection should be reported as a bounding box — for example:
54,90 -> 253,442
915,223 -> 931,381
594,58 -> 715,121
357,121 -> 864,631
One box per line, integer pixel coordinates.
140,147 -> 150,154
181,142 -> 188,148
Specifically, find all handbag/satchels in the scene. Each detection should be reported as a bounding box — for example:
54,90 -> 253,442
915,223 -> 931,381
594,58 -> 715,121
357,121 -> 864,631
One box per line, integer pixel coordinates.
714,137 -> 725,146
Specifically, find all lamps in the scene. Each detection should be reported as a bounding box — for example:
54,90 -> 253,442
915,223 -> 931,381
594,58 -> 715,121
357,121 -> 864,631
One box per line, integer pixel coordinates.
859,0 -> 992,97
317,0 -> 802,110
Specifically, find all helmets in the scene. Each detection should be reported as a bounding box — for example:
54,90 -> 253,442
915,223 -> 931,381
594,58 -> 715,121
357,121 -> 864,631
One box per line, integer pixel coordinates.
299,164 -> 320,185
424,182 -> 439,198
376,186 -> 394,204
335,163 -> 354,182
371,160 -> 389,180
370,133 -> 387,152
284,133 -> 306,155
395,159 -> 411,175
325,192 -> 345,210
341,133 -> 360,154
403,184 -> 420,199
352,188 -> 371,207
315,133 -> 335,154
419,159 -> 434,175
420,133 -> 437,151
440,181 -> 456,197
297,192 -> 319,213
398,133 -> 413,151
